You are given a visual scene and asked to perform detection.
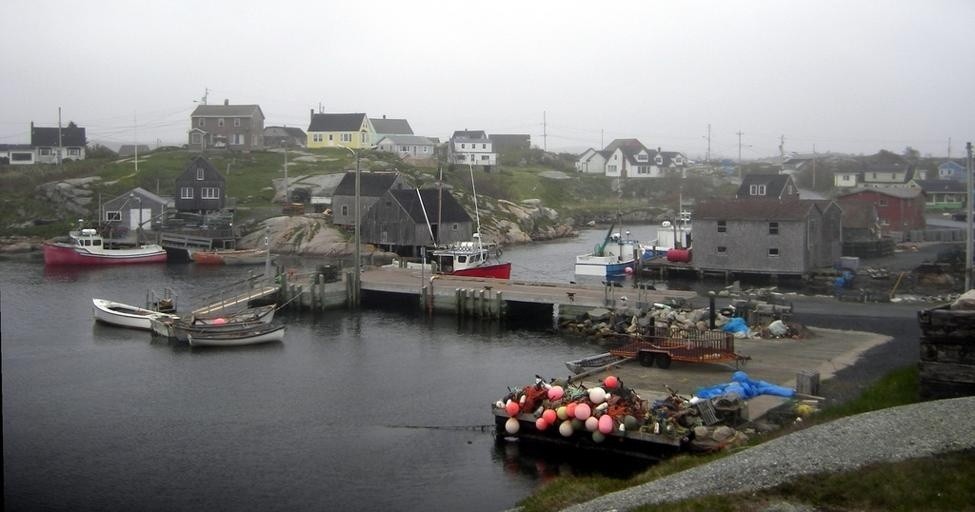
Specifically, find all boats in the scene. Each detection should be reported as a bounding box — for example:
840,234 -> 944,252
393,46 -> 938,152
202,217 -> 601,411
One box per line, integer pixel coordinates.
44,264 -> 164,282
93,289 -> 287,346
43,218 -> 166,265
416,163 -> 512,279
569,201 -> 691,278
184,247 -> 281,266
564,351 -> 623,376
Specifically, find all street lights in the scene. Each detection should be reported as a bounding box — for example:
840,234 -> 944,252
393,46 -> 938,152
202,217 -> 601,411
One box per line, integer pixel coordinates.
336,143 -> 357,275
279,138 -> 292,217
